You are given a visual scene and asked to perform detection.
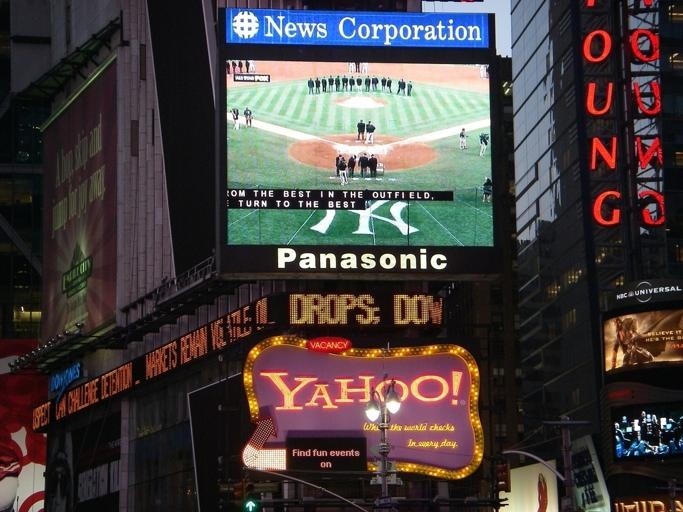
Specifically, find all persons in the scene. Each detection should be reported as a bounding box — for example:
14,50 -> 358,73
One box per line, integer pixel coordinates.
610,317 -> 654,370
536,472 -> 548,512
238,60 -> 244,73
348,61 -> 354,73
366,121 -> 372,138
478,132 -> 488,156
232,60 -> 237,73
459,128 -> 468,149
307,74 -> 412,96
612,408 -> 683,459
356,119 -> 365,140
243,106 -> 253,128
482,178 -> 492,203
245,59 -> 250,73
364,123 -> 376,144
361,63 -> 369,74
335,153 -> 377,186
355,61 -> 360,73
226,60 -> 231,75
229,106 -> 241,131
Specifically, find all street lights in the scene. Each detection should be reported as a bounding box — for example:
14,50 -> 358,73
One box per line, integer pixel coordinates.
353,379 -> 404,512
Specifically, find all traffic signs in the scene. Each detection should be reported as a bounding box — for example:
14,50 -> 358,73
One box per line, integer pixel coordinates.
373,496 -> 391,507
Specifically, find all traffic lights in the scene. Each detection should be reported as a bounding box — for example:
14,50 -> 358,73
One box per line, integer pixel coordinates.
243,499 -> 259,512
495,461 -> 511,492
